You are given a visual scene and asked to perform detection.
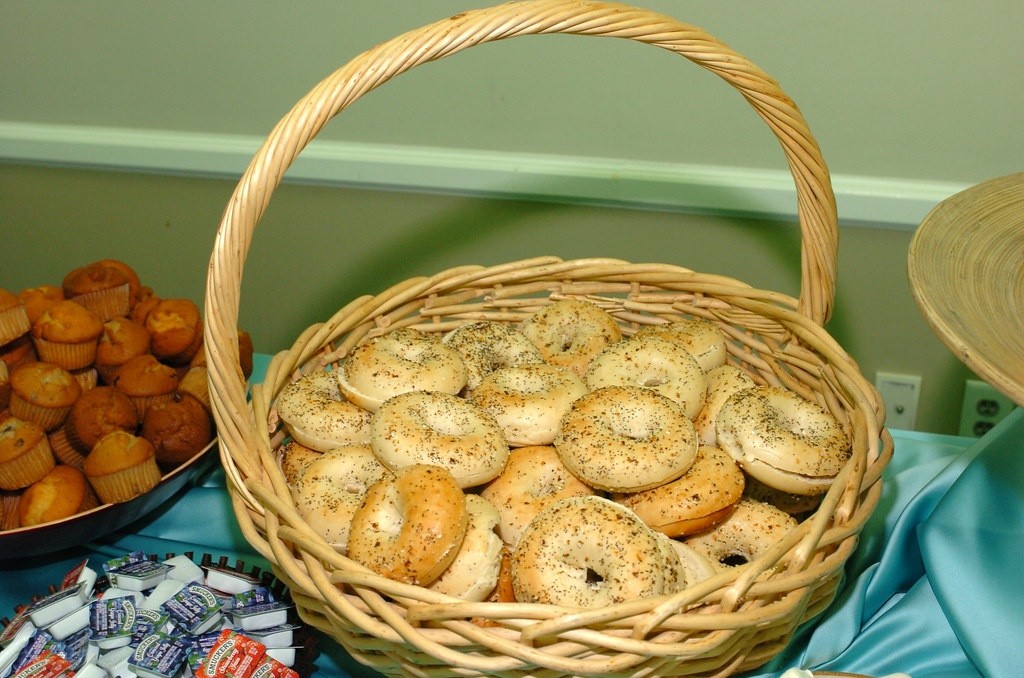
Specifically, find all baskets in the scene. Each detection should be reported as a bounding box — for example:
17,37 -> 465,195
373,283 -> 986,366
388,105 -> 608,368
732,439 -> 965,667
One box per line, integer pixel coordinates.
203,0 -> 895,678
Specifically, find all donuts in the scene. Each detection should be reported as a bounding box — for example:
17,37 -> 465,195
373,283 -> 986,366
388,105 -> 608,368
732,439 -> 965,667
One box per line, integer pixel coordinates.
273,298 -> 850,632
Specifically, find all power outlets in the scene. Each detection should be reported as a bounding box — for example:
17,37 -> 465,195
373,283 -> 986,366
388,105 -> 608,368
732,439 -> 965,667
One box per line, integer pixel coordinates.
957,379 -> 1014,439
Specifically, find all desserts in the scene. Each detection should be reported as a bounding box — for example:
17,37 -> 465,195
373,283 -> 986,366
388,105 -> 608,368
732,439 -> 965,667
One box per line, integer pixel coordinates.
0,256 -> 254,532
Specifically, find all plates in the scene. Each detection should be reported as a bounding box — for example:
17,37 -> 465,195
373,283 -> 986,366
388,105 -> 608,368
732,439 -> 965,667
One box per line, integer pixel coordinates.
908,172 -> 1024,407
0,381 -> 254,562
